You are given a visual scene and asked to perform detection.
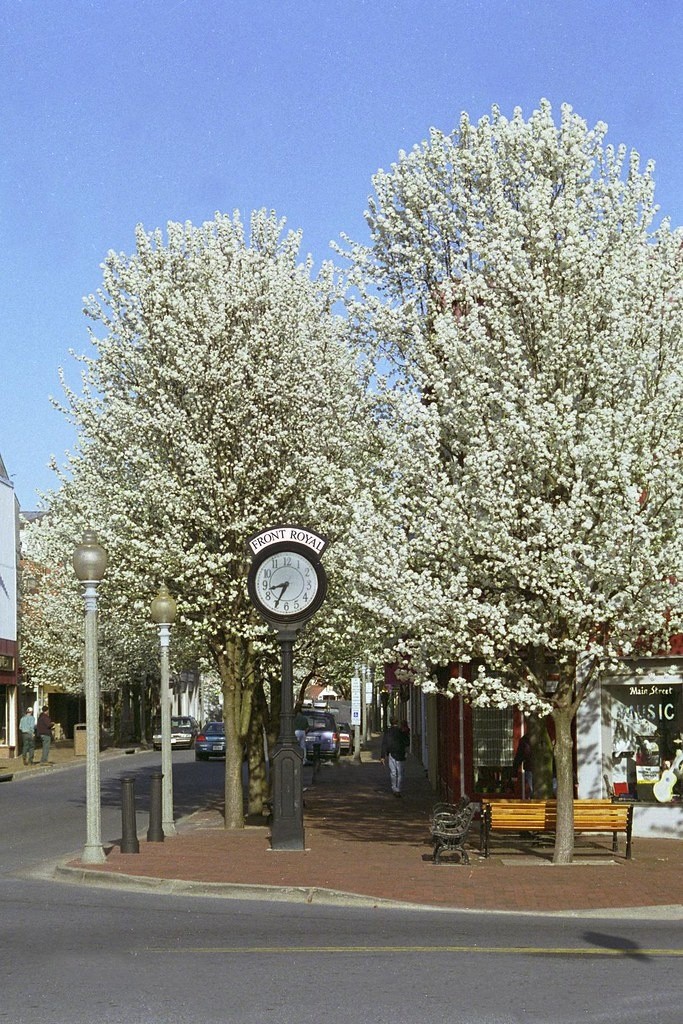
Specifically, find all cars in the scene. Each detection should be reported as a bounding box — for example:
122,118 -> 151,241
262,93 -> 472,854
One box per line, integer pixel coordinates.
152,717 -> 200,749
335,722 -> 351,753
194,722 -> 244,762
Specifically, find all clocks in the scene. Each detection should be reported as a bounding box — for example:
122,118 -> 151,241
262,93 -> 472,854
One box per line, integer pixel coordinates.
248,541 -> 324,623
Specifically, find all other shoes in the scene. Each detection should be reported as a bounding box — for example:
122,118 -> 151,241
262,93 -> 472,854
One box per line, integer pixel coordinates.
40,762 -> 51,766
24,762 -> 35,767
392,790 -> 401,798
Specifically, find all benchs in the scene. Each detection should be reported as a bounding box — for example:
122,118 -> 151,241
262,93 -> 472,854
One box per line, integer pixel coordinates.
481,798 -> 634,860
429,795 -> 479,865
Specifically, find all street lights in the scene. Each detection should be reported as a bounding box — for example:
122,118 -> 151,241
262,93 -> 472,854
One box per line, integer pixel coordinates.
149,578 -> 181,836
72,524 -> 109,863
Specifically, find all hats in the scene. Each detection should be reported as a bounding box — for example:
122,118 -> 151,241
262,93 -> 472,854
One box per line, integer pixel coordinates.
27,707 -> 32,711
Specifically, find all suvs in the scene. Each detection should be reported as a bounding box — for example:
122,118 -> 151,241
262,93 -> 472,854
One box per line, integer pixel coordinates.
300,710 -> 341,762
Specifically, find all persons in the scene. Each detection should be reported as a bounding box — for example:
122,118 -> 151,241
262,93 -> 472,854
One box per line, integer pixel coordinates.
37,706 -> 55,766
19,707 -> 35,766
293,708 -> 310,765
511,734 -> 581,837
381,716 -> 407,798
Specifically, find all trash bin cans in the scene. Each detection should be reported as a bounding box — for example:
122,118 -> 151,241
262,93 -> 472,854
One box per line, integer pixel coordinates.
73,723 -> 87,757
